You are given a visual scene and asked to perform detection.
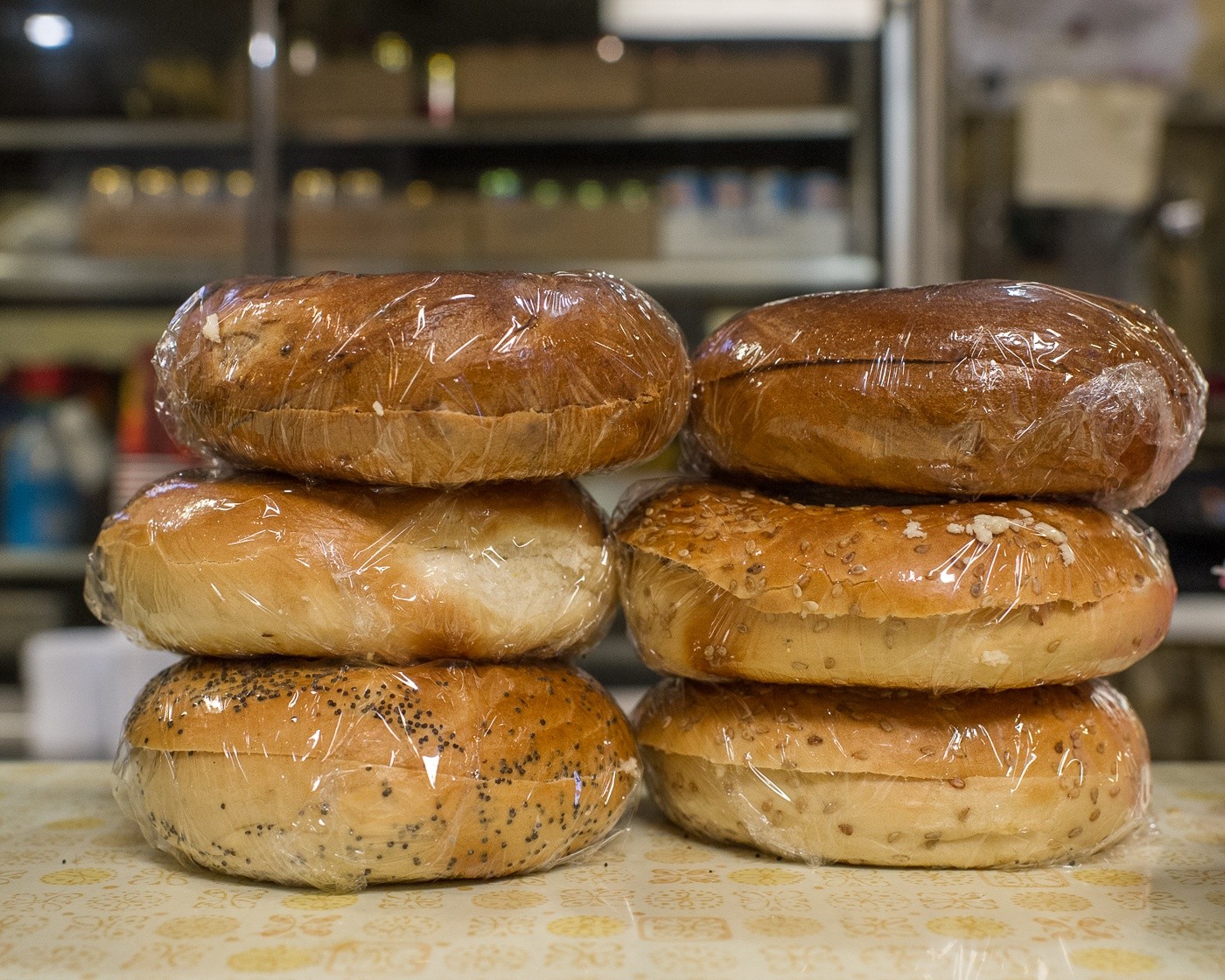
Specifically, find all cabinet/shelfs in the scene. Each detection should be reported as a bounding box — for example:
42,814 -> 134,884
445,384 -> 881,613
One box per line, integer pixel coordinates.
0,0 -> 919,578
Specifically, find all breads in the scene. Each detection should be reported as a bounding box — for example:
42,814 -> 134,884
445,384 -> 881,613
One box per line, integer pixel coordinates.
101,465 -> 622,660
113,655 -> 639,884
607,472 -> 1175,695
149,267 -> 693,489
626,676 -> 1152,867
676,281 -> 1204,501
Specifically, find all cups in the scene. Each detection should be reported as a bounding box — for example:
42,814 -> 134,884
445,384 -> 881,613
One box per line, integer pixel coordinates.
111,344 -> 198,514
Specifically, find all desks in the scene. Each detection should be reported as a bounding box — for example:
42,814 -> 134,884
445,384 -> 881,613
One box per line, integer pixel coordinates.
0,757 -> 1225,980
